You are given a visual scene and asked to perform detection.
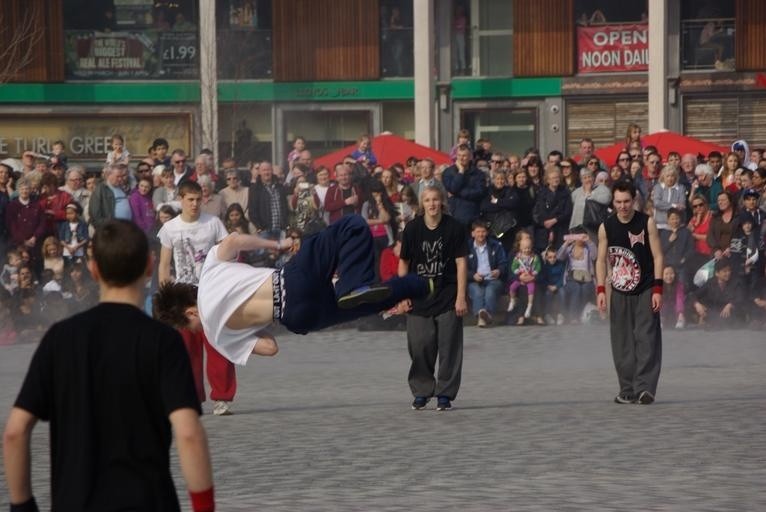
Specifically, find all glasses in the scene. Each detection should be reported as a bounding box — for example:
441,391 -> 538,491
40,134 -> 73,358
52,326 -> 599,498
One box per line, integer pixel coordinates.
0,123 -> 764,255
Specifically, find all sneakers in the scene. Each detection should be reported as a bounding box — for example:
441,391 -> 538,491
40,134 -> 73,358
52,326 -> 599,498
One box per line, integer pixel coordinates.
337,285 -> 394,311
614,393 -> 635,404
477,301 -> 766,332
212,400 -> 232,416
420,275 -> 437,305
411,397 -> 432,410
436,397 -> 453,411
638,390 -> 655,405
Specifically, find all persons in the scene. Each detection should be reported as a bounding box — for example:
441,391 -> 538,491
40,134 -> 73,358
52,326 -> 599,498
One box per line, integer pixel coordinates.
595,176 -> 667,405
589,8 -> 607,26
169,12 -> 200,32
696,10 -> 727,68
379,6 -> 407,76
451,0 -> 470,75
155,178 -> 237,415
575,10 -> 589,30
396,178 -> 471,412
0,216 -> 216,512
1,124 -> 766,344
149,211 -> 435,367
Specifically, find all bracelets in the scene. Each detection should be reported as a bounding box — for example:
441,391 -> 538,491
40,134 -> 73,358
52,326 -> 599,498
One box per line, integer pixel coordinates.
11,495 -> 39,512
188,485 -> 215,512
